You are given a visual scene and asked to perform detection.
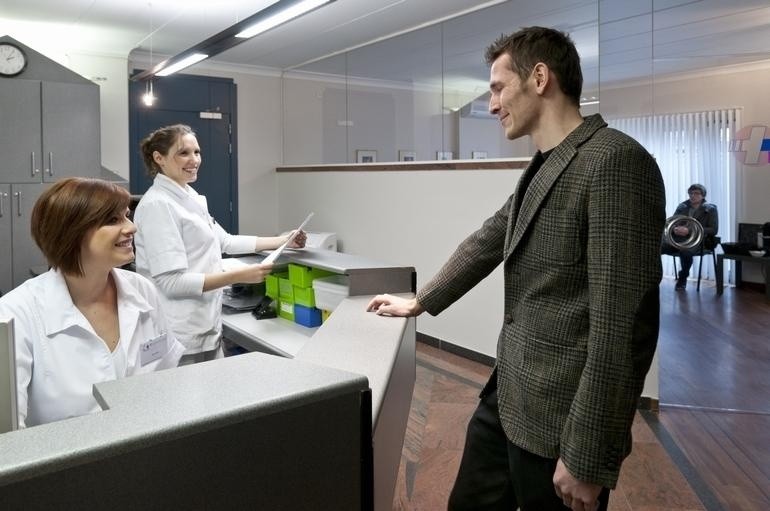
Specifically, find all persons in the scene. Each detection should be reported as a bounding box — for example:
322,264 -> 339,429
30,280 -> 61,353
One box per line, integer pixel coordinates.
0,177 -> 186,429
133,124 -> 307,366
661,184 -> 718,291
366,26 -> 666,511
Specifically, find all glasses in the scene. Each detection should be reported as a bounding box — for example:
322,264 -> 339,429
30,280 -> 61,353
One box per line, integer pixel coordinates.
688,192 -> 703,195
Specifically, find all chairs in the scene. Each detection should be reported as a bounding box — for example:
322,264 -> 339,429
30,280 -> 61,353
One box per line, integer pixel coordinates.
670,221 -> 770,298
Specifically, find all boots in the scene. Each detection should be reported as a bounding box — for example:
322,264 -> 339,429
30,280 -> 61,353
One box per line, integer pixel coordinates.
675,271 -> 689,291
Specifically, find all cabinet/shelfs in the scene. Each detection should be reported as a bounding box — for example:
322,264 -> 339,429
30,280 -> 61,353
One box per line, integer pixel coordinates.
0,184 -> 49,292
1,82 -> 103,184
